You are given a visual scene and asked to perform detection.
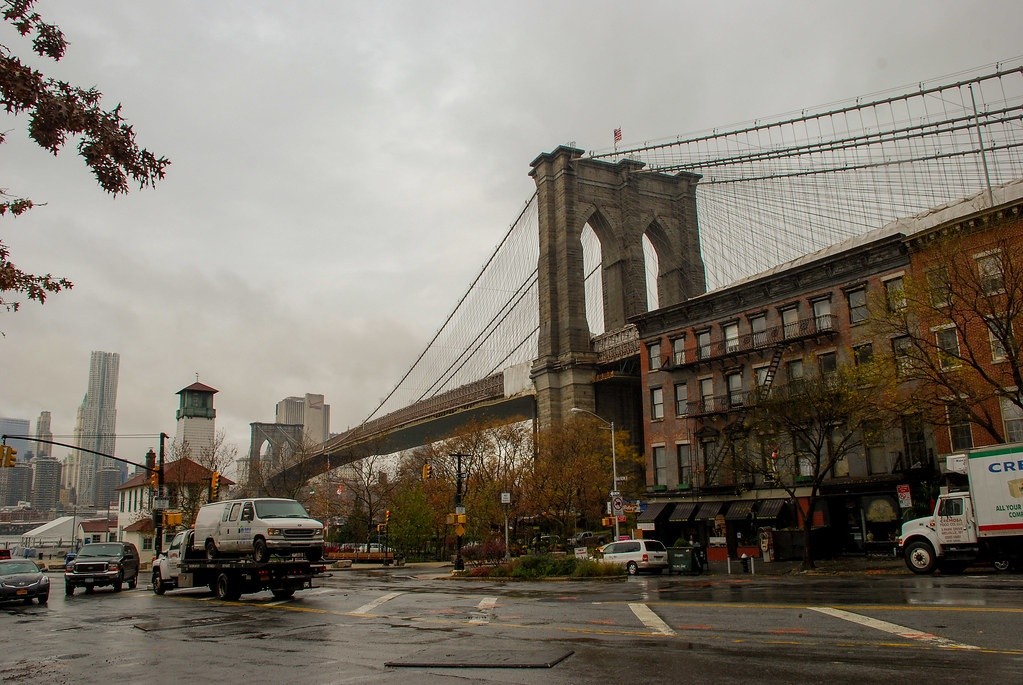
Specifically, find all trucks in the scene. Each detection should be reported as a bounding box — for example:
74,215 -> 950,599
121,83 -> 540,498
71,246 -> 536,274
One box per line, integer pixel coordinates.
899,441 -> 1023,576
152,529 -> 338,601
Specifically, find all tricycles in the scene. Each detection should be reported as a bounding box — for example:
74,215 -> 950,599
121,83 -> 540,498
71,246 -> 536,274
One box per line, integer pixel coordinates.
64,553 -> 77,566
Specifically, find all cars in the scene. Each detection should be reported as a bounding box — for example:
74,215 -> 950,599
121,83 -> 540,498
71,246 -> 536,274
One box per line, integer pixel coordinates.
325,542 -> 394,554
0,559 -> 50,603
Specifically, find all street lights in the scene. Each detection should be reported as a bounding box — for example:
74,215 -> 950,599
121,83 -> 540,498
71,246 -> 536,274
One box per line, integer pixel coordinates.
571,407 -> 619,541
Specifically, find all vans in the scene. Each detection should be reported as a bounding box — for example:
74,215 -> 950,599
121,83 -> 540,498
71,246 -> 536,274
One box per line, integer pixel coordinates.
532,535 -> 560,545
194,498 -> 325,564
64,542 -> 140,595
588,539 -> 669,576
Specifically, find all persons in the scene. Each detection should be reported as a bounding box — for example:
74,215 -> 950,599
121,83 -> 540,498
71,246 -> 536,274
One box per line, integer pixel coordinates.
866,528 -> 874,540
891,528 -> 900,541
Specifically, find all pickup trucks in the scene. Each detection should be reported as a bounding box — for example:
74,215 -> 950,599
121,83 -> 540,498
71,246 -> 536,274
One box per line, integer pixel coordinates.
577,531 -> 604,541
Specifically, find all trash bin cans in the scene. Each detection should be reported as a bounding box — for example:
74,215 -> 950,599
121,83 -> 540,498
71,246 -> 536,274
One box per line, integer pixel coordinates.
39,553 -> 43,560
666,545 -> 693,574
393,552 -> 403,566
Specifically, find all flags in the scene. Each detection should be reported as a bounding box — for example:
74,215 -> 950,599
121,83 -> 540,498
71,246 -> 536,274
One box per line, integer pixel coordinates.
614,126 -> 622,142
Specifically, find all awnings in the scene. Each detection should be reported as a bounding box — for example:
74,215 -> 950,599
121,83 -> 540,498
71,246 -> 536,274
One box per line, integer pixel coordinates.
757,499 -> 784,519
725,501 -> 755,520
668,502 -> 696,521
638,503 -> 667,520
694,502 -> 723,521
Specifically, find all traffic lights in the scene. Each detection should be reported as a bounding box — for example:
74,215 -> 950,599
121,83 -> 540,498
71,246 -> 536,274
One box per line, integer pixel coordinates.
211,472 -> 222,499
423,464 -> 432,480
0,445 -> 17,468
386,511 -> 391,522
